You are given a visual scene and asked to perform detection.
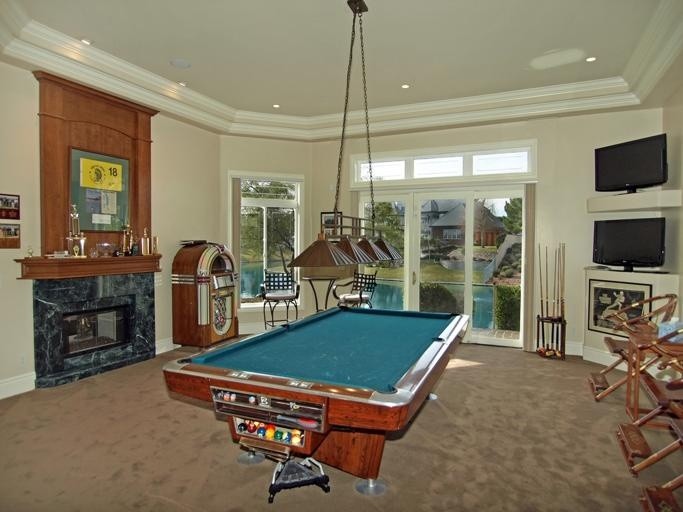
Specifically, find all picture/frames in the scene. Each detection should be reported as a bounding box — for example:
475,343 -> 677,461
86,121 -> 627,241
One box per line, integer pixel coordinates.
0,223 -> 22,250
586,277 -> 653,339
0,192 -> 21,220
67,146 -> 132,235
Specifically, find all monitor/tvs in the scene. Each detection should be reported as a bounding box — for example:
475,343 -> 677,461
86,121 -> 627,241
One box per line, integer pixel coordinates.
592,217 -> 665,272
594,133 -> 667,193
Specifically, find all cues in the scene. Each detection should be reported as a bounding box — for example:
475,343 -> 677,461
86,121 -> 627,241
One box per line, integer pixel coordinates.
543,245 -> 550,350
538,243 -> 545,349
550,250 -> 557,349
555,248 -> 559,351
560,242 -> 566,361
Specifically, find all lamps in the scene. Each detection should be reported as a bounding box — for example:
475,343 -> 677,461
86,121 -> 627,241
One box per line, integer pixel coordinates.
356,235 -> 392,261
335,234 -> 375,264
375,236 -> 405,261
286,232 -> 359,268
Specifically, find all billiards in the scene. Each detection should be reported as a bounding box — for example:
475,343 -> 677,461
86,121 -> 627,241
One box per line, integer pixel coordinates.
239,419 -> 301,445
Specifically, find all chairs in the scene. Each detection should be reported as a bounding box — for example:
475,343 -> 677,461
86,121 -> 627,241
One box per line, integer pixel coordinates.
586,292 -> 679,403
612,353 -> 683,480
332,267 -> 379,309
637,474 -> 683,512
255,268 -> 301,331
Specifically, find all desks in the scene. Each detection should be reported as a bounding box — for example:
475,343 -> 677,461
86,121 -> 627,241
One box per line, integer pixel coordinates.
161,305 -> 471,498
302,276 -> 339,313
624,336 -> 683,432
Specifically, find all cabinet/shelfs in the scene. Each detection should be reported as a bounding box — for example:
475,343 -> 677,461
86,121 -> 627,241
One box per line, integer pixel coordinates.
171,240 -> 240,349
581,188 -> 683,386
13,68 -> 163,390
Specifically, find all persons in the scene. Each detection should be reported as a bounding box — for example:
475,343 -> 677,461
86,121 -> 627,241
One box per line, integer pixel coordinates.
606,291 -> 625,312
